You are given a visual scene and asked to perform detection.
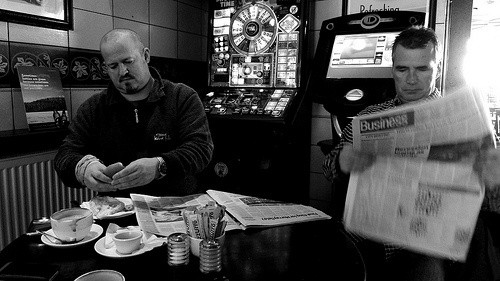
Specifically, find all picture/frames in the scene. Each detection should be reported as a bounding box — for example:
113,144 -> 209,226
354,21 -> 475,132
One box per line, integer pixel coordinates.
0,0 -> 74,31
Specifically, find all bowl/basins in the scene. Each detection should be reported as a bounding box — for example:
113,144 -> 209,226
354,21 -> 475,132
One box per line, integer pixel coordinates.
190,230 -> 225,257
74,269 -> 125,281
113,231 -> 142,254
50,208 -> 93,243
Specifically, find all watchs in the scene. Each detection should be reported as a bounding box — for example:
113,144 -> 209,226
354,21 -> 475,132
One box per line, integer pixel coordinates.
157,157 -> 167,180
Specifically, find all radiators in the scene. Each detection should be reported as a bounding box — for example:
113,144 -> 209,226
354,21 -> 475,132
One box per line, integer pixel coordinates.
0,150 -> 99,251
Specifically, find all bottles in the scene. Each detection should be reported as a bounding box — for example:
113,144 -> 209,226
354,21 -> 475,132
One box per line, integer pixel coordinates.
167,233 -> 190,266
199,238 -> 221,273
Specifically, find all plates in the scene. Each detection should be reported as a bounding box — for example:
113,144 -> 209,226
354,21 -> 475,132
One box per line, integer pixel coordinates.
80,197 -> 134,219
93,231 -> 158,258
40,224 -> 103,247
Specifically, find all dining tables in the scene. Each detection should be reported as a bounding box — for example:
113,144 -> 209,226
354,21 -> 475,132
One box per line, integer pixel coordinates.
0,197 -> 366,281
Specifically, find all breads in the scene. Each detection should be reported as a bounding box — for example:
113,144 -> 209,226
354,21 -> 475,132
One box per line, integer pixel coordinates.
103,162 -> 123,179
89,196 -> 133,220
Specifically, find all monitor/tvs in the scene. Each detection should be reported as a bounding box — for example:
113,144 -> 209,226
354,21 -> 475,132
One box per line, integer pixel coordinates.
324,29 -> 404,83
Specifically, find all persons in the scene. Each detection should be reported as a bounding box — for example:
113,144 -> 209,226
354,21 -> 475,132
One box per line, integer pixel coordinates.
320,24 -> 500,281
55,28 -> 214,197
